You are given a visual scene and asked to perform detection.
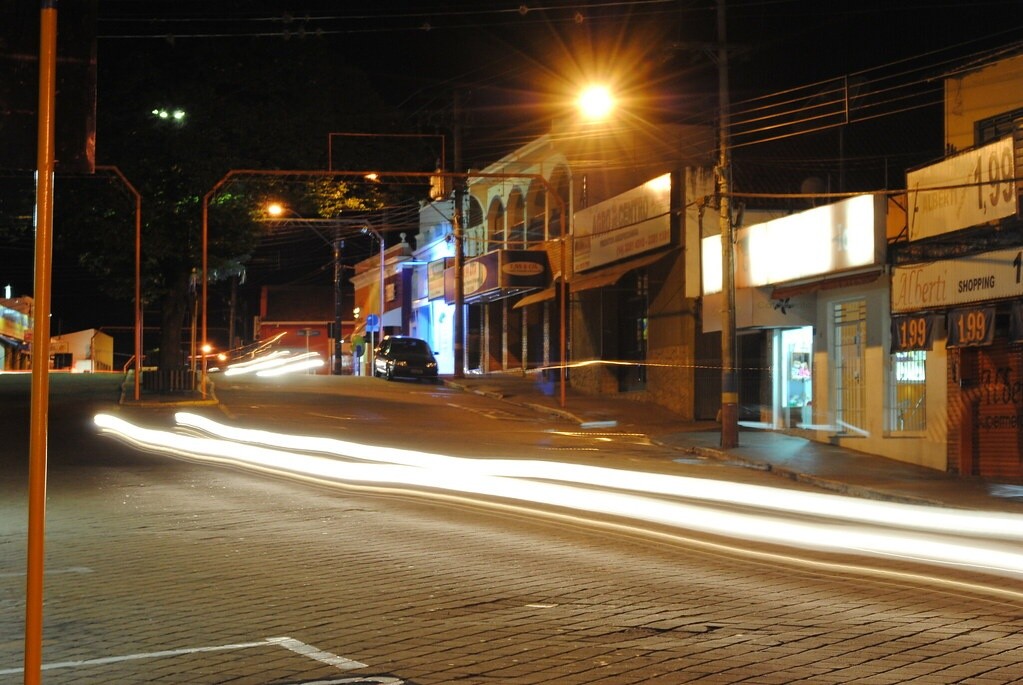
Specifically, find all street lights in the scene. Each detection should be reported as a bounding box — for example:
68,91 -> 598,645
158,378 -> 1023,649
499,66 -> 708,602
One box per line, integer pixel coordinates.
577,86 -> 739,453
267,202 -> 341,374
365,174 -> 465,380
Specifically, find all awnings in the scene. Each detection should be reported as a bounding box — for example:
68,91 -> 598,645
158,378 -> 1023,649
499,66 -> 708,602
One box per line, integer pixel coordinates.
0,334 -> 28,360
350,316 -> 379,342
342,334 -> 351,343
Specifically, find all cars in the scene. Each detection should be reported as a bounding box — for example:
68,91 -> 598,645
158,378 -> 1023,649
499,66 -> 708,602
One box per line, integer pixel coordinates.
374,335 -> 440,383
